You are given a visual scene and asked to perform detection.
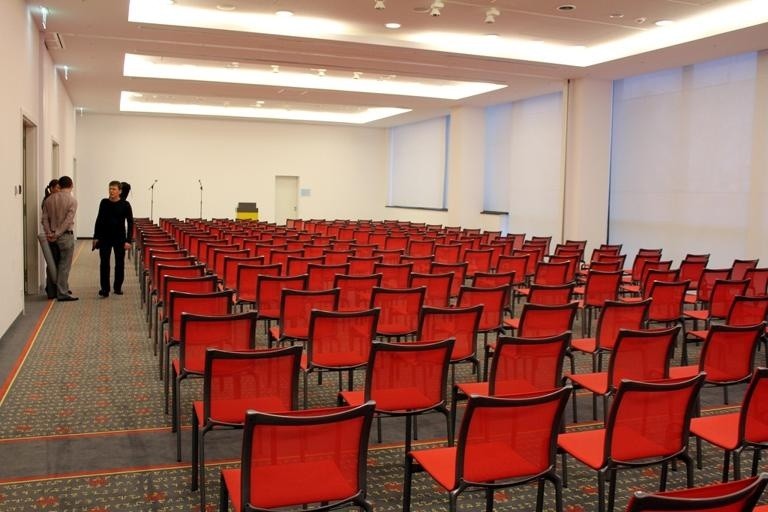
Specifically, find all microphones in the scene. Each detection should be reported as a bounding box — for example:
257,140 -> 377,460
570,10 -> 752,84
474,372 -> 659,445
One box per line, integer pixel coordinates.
198,179 -> 202,188
149,179 -> 158,190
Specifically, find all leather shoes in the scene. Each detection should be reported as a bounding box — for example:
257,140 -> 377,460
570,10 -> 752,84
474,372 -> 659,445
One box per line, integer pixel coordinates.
114,288 -> 123,295
99,289 -> 109,297
58,294 -> 79,301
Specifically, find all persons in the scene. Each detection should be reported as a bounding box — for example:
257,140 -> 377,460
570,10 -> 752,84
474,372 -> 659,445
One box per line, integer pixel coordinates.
38,178 -> 75,299
90,180 -> 134,298
40,176 -> 80,302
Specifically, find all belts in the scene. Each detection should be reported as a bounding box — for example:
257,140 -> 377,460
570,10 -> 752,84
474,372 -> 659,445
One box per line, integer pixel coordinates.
65,231 -> 73,235
